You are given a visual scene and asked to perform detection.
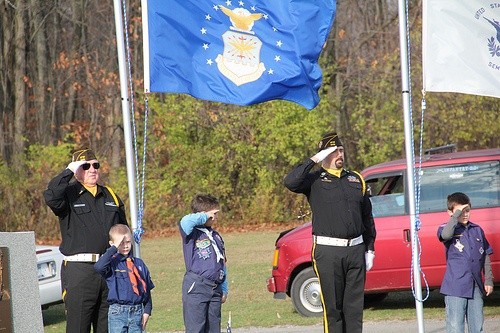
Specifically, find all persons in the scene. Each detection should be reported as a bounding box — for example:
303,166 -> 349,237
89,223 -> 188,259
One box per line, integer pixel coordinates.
178,193 -> 228,333
283,131 -> 377,333
93,223 -> 155,333
436,192 -> 494,333
44,149 -> 129,333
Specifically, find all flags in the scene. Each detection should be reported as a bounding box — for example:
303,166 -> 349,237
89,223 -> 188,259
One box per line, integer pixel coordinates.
421,0 -> 500,98
141,0 -> 337,110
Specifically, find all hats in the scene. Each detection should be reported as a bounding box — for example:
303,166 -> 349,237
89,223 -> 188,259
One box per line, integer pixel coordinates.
318,132 -> 343,152
70,145 -> 97,161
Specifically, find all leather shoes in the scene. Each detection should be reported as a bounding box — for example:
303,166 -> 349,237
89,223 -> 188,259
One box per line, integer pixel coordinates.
312,234 -> 364,246
63,253 -> 103,262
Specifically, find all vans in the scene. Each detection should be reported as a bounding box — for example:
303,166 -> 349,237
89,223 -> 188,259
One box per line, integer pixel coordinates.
267,146 -> 499,317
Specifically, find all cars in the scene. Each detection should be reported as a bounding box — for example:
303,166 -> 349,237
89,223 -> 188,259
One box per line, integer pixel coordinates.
34,244 -> 64,307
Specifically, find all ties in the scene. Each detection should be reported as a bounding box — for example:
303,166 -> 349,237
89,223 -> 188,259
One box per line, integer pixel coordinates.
195,227 -> 224,263
126,258 -> 147,295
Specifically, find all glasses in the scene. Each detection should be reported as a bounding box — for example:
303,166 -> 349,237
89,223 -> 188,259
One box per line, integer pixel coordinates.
80,163 -> 100,170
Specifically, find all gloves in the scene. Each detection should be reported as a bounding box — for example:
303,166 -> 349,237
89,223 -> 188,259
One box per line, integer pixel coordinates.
311,146 -> 338,163
66,161 -> 87,173
365,249 -> 375,271
